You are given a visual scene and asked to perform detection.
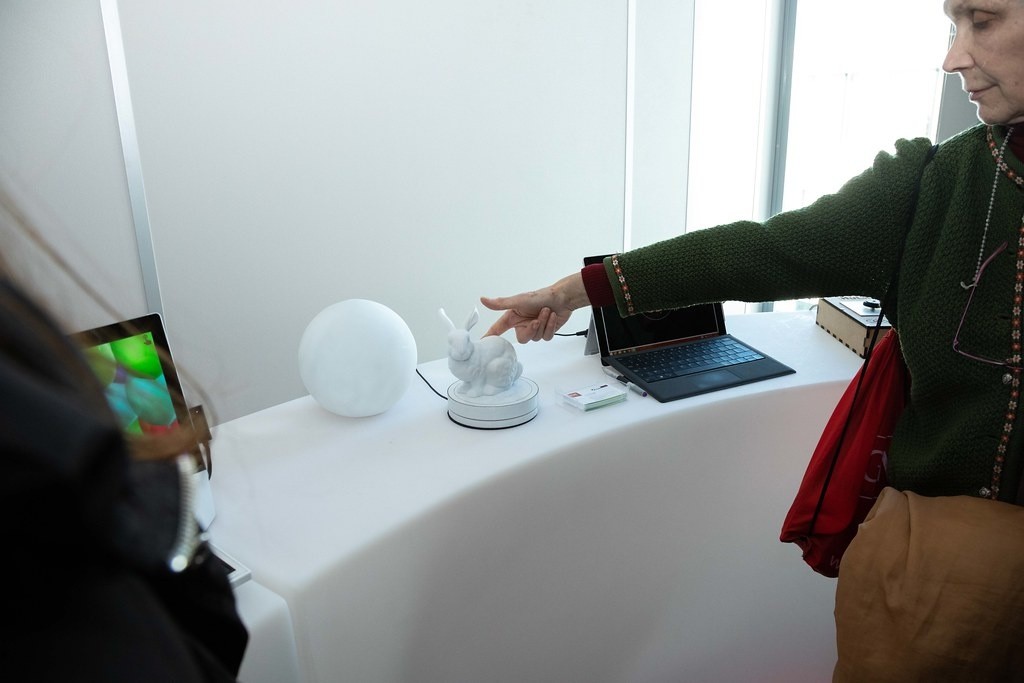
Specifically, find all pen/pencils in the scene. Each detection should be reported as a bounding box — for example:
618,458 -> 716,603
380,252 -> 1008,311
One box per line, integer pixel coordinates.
602,367 -> 647,396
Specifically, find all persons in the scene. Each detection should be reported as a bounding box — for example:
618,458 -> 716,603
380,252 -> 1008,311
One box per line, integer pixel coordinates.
0,189 -> 252,683
480,0 -> 1024,683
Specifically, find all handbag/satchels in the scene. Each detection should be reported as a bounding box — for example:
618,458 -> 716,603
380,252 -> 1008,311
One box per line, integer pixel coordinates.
781,327 -> 911,580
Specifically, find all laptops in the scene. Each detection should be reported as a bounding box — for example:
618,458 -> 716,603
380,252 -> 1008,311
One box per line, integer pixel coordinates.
584,255 -> 796,404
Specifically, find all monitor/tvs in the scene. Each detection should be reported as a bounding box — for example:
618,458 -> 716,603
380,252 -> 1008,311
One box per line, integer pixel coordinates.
67,313 -> 220,537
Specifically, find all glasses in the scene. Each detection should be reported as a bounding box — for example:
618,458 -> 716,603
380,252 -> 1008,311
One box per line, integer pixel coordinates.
950,239 -> 1022,376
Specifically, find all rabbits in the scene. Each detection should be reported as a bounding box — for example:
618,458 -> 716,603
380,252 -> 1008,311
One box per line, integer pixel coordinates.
439,307 -> 523,398
